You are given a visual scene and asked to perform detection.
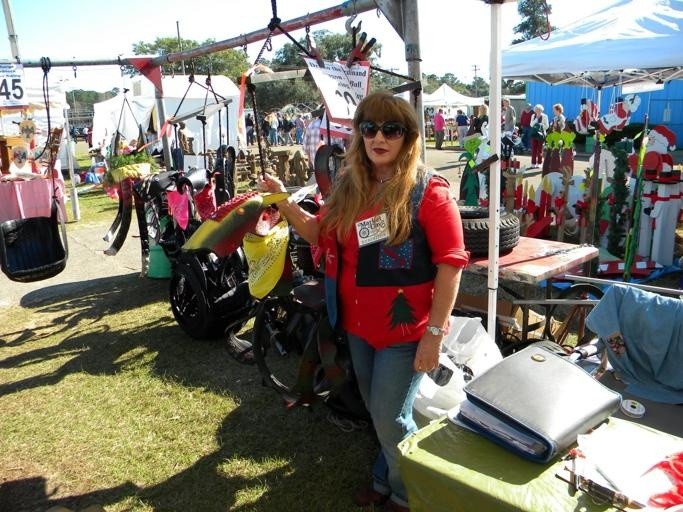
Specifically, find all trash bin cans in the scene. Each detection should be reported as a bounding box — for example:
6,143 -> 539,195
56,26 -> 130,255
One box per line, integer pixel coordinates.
148,246 -> 173,278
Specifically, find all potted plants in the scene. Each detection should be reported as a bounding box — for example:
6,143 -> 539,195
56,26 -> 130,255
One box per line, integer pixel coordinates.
108,152 -> 159,181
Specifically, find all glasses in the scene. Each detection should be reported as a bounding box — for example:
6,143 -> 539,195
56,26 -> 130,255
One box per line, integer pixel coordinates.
359,121 -> 407,140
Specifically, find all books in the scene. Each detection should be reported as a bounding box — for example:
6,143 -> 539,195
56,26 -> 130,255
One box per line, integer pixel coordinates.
447,343 -> 623,465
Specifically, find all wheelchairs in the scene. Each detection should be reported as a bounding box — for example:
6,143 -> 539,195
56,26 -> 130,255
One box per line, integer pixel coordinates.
252,240 -> 351,412
157,210 -> 260,340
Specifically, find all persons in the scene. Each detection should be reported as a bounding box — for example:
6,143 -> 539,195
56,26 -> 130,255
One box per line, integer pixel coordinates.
128,138 -> 137,151
257,91 -> 473,511
245,111 -> 310,147
177,121 -> 190,158
71,123 -> 89,144
433,98 -> 566,169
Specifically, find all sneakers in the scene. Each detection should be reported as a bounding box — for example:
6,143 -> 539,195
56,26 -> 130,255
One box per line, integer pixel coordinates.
355,489 -> 409,512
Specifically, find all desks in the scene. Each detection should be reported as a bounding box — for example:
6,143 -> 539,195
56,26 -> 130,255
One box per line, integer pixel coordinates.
466,234 -> 601,350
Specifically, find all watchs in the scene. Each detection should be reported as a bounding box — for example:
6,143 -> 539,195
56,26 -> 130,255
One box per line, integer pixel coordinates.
426,326 -> 445,336
